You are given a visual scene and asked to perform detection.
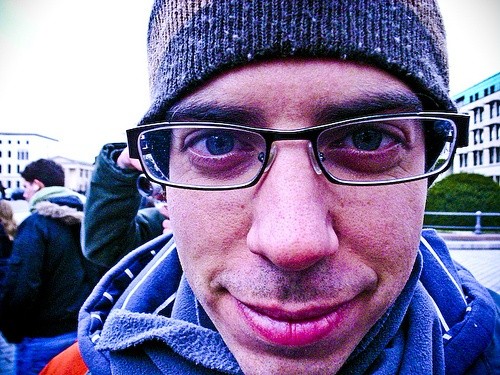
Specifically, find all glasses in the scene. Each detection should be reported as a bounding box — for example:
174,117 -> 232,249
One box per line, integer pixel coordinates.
126,112 -> 470,190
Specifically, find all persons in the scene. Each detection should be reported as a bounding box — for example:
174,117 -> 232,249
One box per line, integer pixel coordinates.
39,0 -> 500,375
0,142 -> 173,345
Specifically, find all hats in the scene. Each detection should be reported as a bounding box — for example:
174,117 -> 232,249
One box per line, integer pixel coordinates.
137,0 -> 457,188
